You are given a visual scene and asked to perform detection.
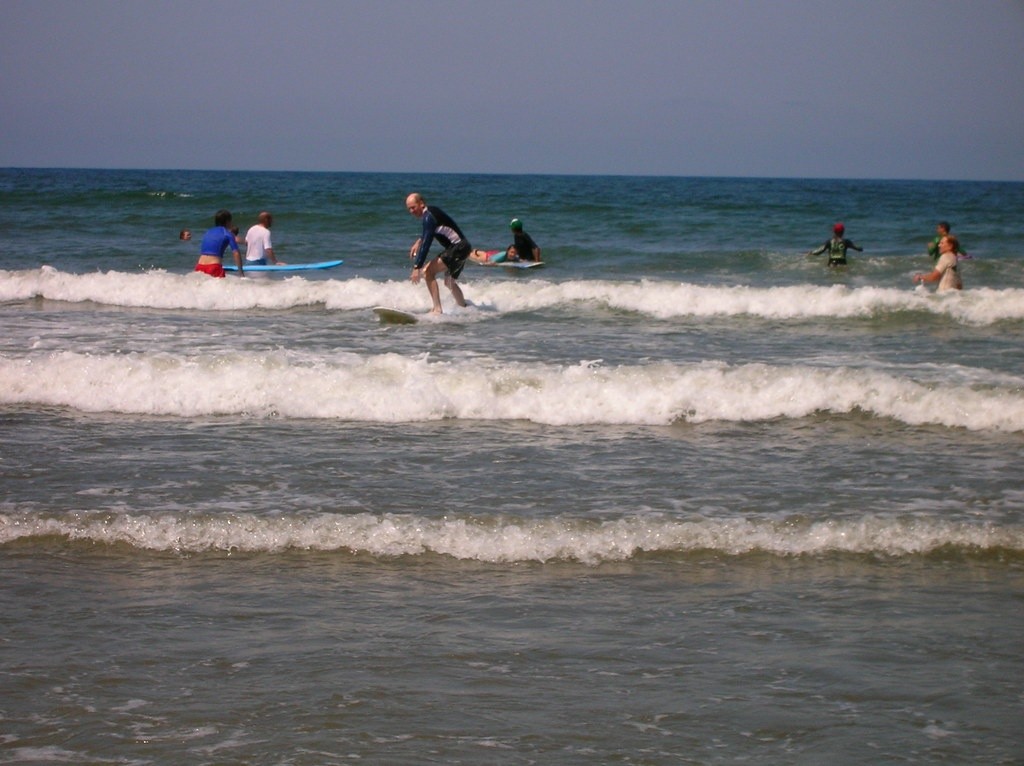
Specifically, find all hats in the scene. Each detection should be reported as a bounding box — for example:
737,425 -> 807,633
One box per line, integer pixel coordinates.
508,218 -> 522,229
833,224 -> 844,233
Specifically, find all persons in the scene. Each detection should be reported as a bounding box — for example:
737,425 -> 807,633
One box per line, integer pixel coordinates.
406,193 -> 473,313
180,229 -> 191,240
811,223 -> 864,267
194,209 -> 286,278
470,218 -> 540,265
913,222 -> 969,293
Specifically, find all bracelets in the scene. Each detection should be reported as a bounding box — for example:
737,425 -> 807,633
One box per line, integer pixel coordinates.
414,265 -> 419,269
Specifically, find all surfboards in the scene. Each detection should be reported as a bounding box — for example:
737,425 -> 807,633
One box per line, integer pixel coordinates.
466,255 -> 545,268
222,259 -> 344,272
372,307 -> 418,327
